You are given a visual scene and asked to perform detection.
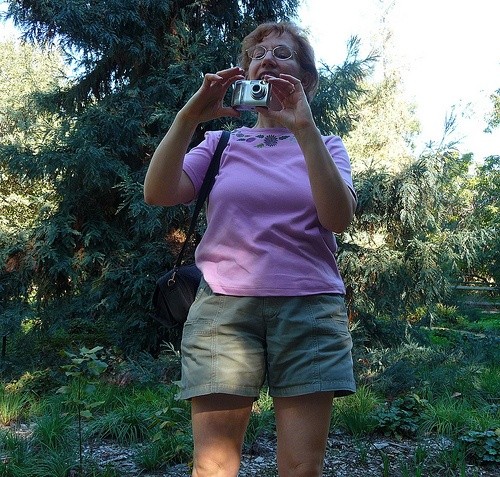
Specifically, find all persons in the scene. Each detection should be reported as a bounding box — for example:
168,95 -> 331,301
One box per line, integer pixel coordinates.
144,21 -> 357,477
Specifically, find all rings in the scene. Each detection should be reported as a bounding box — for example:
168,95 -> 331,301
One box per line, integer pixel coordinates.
289,88 -> 295,95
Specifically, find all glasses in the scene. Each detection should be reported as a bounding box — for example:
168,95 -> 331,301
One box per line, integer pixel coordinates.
246,45 -> 298,61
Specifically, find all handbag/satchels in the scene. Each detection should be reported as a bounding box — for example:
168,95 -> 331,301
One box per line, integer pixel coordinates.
149,265 -> 203,325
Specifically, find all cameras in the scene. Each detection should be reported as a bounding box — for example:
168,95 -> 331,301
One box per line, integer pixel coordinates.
231,79 -> 272,110
